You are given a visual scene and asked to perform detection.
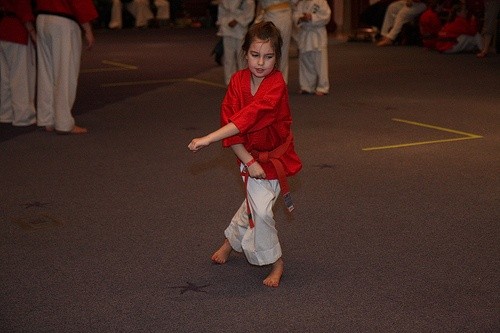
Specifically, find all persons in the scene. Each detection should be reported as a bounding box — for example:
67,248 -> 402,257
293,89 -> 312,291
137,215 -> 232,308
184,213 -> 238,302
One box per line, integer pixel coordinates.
361,0 -> 500,56
108,0 -> 169,30
187,21 -> 302,288
210,0 -> 332,95
1,0 -> 98,134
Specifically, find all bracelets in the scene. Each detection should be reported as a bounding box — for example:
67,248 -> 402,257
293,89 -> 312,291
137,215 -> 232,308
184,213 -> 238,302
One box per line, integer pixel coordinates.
244,158 -> 256,168
